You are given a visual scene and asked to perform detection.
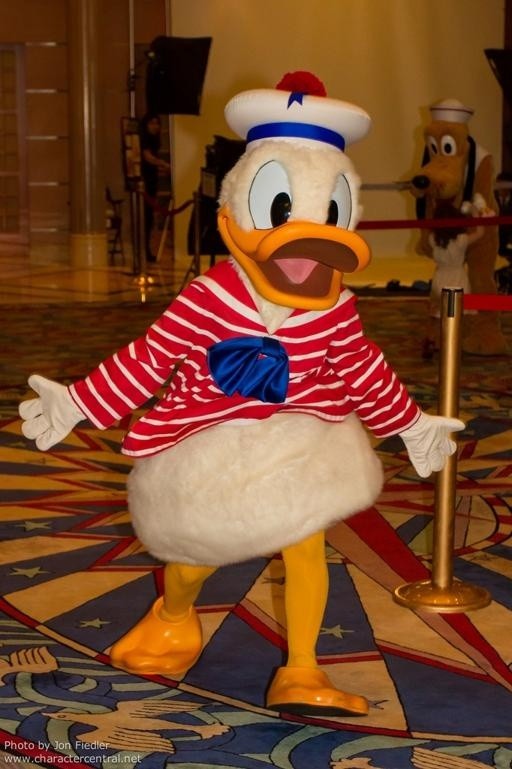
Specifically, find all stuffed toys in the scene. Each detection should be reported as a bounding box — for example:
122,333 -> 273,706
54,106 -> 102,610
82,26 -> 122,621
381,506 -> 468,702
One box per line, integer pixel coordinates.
410,98 -> 500,319
18,70 -> 467,717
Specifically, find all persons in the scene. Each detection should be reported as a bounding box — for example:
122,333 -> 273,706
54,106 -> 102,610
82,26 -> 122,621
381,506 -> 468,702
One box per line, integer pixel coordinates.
423,203 -> 486,320
140,113 -> 170,263
